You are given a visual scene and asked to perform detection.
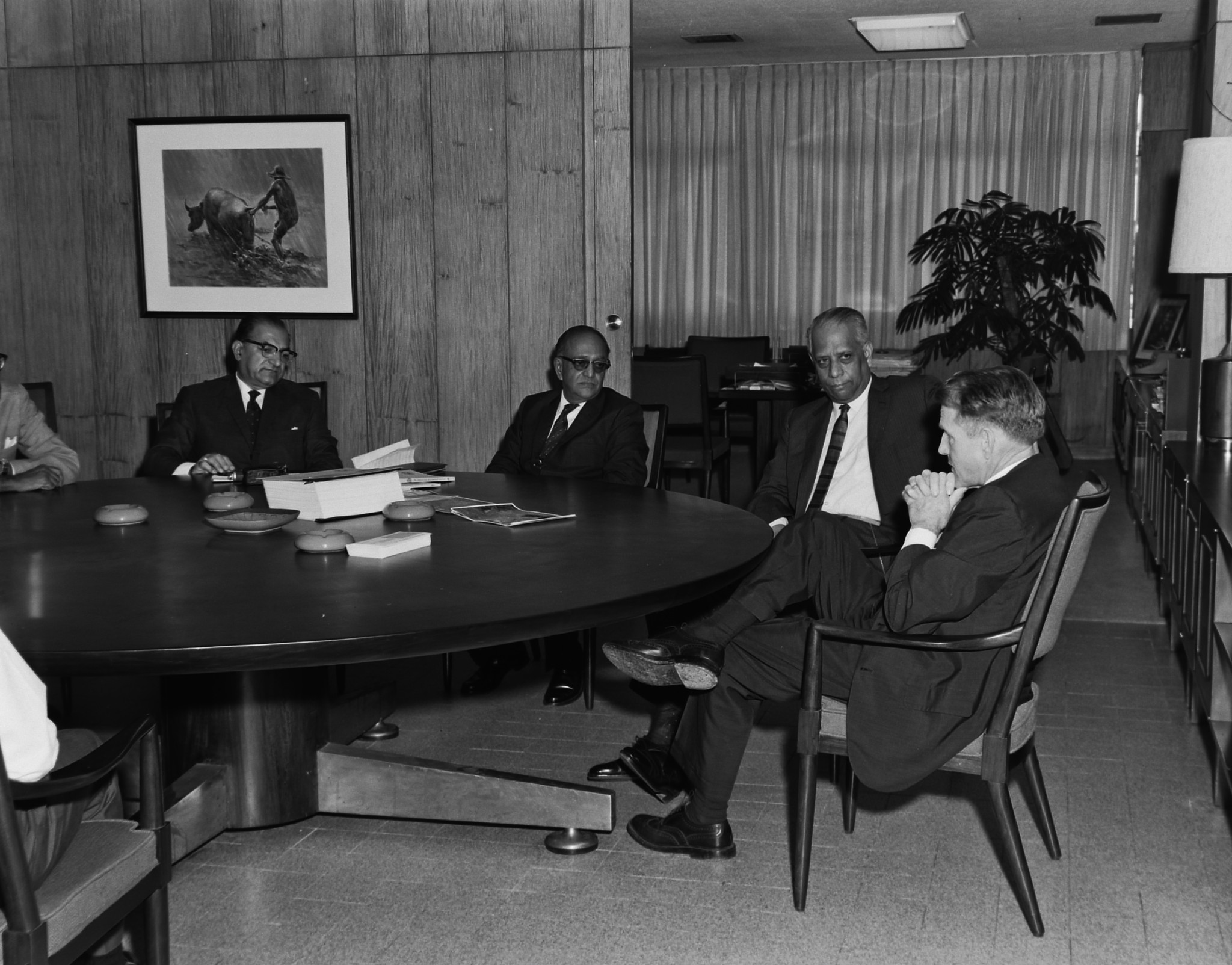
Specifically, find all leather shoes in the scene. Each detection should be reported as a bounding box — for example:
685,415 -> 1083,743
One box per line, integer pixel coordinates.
627,802 -> 736,860
618,748 -> 684,803
359,720 -> 401,739
586,758 -> 632,781
460,651 -> 510,696
603,635 -> 725,689
543,642 -> 585,706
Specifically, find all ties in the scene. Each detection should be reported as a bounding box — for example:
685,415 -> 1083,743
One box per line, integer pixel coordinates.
246,390 -> 262,452
808,403 -> 850,514
533,403 -> 579,469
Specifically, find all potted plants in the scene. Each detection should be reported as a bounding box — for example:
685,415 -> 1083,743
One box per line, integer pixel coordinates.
896,191 -> 1116,379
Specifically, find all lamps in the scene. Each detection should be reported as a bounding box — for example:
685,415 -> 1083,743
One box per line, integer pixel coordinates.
1168,137 -> 1231,439
847,11 -> 974,51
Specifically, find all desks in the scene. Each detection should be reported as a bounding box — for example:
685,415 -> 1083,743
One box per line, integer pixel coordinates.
0,468 -> 774,867
717,364 -> 823,488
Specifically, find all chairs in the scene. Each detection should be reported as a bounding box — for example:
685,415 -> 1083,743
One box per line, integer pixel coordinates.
0,709 -> 176,965
633,353 -> 732,503
793,469 -> 1111,939
444,404 -> 672,710
683,335 -> 771,483
156,380 -> 327,438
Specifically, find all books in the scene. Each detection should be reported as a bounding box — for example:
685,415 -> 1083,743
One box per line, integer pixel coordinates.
261,462 -> 455,523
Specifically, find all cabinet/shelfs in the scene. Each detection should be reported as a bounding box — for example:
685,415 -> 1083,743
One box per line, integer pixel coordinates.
1110,354 -> 1232,831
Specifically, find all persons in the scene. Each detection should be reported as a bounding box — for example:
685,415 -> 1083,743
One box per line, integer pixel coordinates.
143,315 -> 341,480
0,628 -> 124,905
587,307 -> 944,802
459,325 -> 648,705
601,366 -> 1074,859
0,354 -> 81,493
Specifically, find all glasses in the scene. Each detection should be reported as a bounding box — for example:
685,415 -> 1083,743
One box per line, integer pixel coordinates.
240,338 -> 297,364
558,355 -> 611,373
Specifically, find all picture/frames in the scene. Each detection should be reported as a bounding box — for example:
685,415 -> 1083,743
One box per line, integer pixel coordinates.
127,114 -> 358,320
1130,292 -> 1191,363
